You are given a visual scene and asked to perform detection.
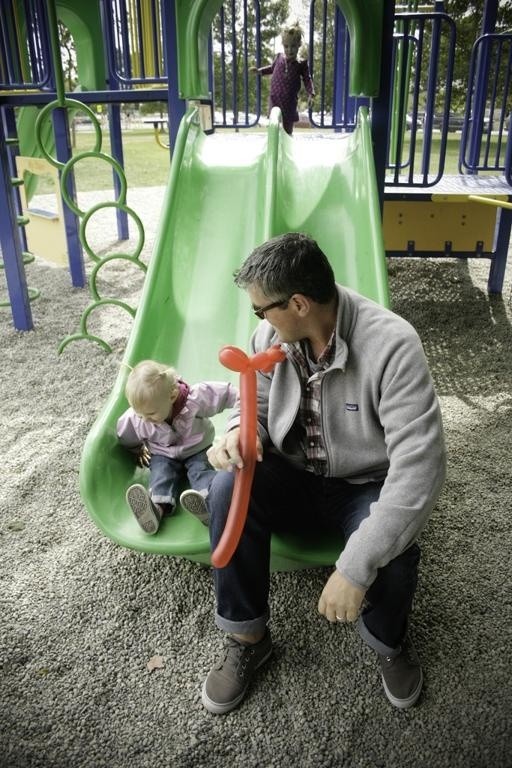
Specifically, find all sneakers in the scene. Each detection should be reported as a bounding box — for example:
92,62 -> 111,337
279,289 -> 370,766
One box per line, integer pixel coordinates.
126,484 -> 160,536
179,490 -> 210,526
201,626 -> 272,715
380,634 -> 422,709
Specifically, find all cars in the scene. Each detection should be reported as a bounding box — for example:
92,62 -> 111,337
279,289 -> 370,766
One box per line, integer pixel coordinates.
406,111 -> 493,133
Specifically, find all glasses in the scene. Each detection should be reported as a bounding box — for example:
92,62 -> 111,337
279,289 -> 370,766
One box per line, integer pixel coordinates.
251,297 -> 286,321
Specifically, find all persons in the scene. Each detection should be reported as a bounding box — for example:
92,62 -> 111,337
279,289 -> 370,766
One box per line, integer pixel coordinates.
202,232 -> 446,714
114,360 -> 238,534
247,20 -> 317,136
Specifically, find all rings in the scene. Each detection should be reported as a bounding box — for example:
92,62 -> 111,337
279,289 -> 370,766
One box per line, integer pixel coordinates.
336,616 -> 344,620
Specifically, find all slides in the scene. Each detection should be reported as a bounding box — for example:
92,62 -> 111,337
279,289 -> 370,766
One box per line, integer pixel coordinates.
79,101 -> 395,576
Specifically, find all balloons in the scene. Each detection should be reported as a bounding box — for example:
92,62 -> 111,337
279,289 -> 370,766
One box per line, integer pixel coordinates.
211,344 -> 284,568
